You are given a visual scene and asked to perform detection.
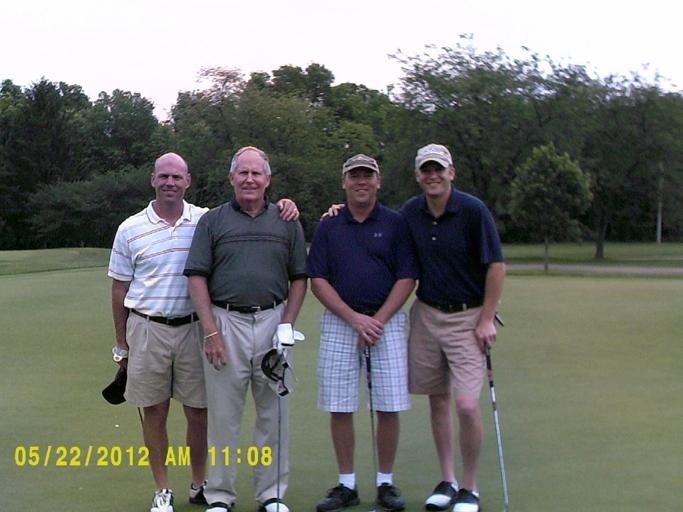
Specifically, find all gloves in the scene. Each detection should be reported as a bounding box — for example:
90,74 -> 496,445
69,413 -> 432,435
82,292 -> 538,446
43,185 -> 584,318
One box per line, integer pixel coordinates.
272,323 -> 307,358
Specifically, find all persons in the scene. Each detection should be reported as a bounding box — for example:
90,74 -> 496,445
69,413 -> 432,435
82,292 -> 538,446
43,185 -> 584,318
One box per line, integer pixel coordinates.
183,145 -> 309,512
321,143 -> 507,512
107,152 -> 299,512
308,154 -> 421,512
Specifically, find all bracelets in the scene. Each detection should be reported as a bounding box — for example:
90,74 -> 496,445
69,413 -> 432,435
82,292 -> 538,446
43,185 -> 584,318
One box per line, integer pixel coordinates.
203,331 -> 220,340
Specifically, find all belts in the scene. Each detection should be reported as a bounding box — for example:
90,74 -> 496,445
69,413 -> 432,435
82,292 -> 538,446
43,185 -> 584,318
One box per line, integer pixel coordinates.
357,310 -> 377,316
130,308 -> 199,327
419,298 -> 483,314
211,298 -> 284,314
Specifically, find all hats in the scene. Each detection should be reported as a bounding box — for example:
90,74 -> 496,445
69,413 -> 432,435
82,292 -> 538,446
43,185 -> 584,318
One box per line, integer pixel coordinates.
343,154 -> 380,174
260,350 -> 297,397
101,366 -> 129,405
415,144 -> 453,169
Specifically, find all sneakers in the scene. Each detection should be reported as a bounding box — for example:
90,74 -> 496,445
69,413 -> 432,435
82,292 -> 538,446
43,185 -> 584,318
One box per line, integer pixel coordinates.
316,484 -> 359,511
150,490 -> 174,512
189,480 -> 208,503
259,498 -> 290,512
205,502 -> 229,512
425,482 -> 458,510
376,484 -> 406,511
452,489 -> 481,512
